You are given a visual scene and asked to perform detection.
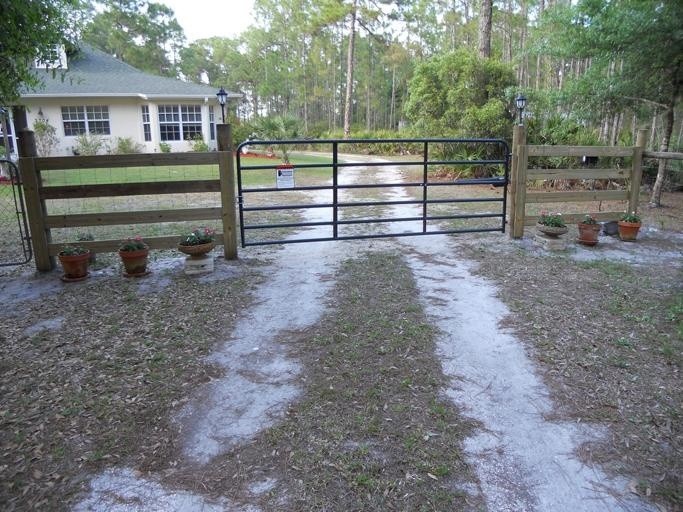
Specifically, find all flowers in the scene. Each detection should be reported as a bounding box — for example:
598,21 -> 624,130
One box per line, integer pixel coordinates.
582,210 -> 601,225
61,245 -> 88,256
177,223 -> 217,246
118,235 -> 147,253
537,209 -> 565,227
620,207 -> 642,223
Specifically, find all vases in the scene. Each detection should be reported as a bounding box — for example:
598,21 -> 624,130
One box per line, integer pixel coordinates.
177,239 -> 216,276
118,246 -> 150,277
57,251 -> 92,281
617,221 -> 641,242
533,221 -> 569,252
575,221 -> 602,247
